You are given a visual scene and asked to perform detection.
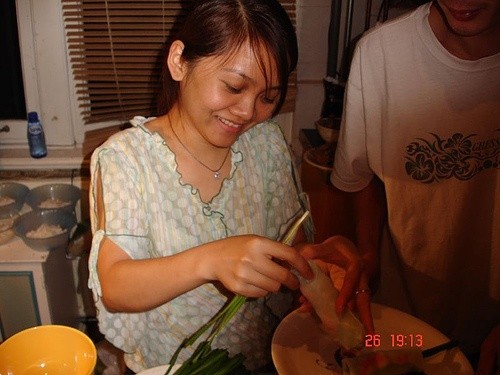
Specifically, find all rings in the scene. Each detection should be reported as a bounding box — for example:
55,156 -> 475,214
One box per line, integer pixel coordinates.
353,288 -> 369,295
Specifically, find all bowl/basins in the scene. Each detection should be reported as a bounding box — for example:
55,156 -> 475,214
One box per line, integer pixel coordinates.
0,183 -> 83,251
316,118 -> 341,143
0,325 -> 97,375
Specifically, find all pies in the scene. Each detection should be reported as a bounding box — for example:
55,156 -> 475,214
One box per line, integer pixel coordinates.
290,260 -> 374,354
341,344 -> 425,375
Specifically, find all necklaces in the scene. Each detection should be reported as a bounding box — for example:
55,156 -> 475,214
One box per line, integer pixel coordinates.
167,112 -> 231,178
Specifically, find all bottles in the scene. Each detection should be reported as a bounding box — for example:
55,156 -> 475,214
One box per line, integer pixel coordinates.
27,112 -> 47,158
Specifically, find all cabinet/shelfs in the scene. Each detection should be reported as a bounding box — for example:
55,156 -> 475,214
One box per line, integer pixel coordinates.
0,240 -> 81,346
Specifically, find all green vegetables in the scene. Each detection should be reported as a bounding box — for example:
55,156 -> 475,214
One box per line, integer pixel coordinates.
166,209 -> 310,375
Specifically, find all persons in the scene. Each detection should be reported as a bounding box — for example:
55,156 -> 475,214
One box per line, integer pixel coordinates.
88,0 -> 376,375
330,0 -> 500,375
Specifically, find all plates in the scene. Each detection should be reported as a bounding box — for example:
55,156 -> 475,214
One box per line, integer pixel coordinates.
304,148 -> 334,170
270,302 -> 474,375
